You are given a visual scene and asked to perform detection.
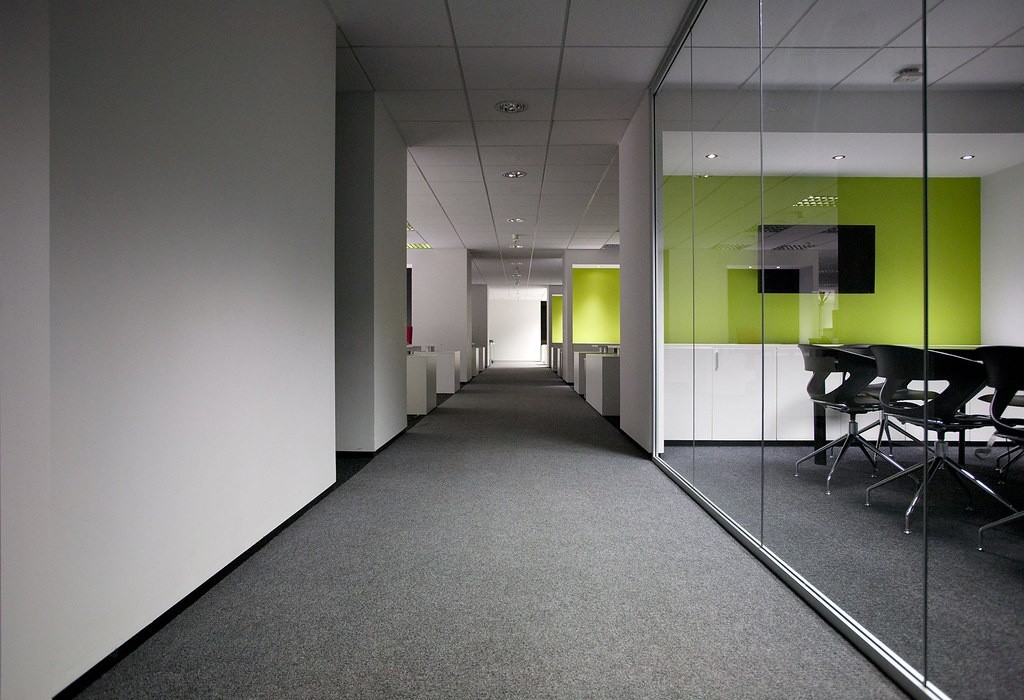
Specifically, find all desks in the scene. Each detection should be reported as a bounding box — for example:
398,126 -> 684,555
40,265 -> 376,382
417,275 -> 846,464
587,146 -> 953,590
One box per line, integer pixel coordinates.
814,345 -> 1024,465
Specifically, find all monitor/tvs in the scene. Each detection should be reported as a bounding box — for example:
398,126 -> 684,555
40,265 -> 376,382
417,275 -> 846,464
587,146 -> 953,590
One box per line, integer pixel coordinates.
757,225 -> 876,294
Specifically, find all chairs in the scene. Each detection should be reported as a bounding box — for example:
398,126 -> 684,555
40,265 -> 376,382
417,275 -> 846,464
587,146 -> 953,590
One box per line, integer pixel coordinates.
864,344 -> 1018,535
975,345 -> 1024,552
795,343 -> 920,496
